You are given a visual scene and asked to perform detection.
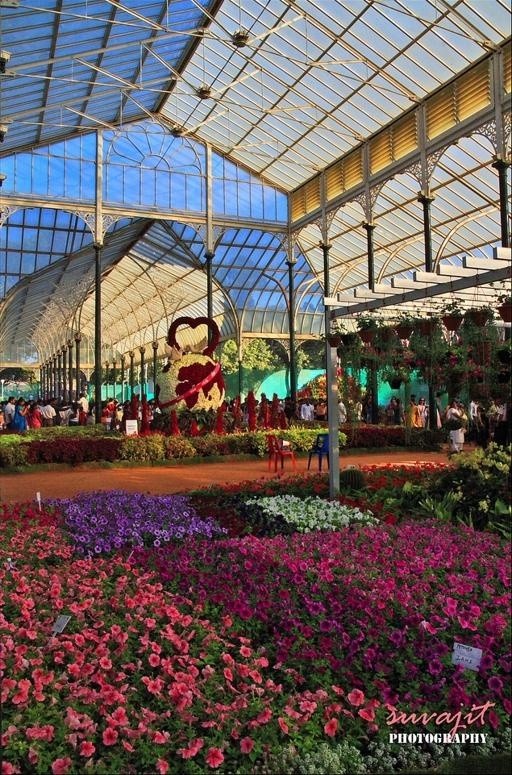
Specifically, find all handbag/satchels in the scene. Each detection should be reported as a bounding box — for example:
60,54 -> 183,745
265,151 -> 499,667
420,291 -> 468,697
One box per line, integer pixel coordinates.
10,412 -> 25,430
445,421 -> 463,430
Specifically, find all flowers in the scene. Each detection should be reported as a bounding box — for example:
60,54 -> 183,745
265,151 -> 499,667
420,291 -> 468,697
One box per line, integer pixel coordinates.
326,290 -> 512,401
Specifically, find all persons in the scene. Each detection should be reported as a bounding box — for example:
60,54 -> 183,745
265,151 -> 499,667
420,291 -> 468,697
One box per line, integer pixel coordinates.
4,393 -> 511,431
447,421 -> 467,455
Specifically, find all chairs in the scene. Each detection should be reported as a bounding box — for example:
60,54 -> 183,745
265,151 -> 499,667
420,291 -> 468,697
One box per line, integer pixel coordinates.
265,433 -> 329,472
